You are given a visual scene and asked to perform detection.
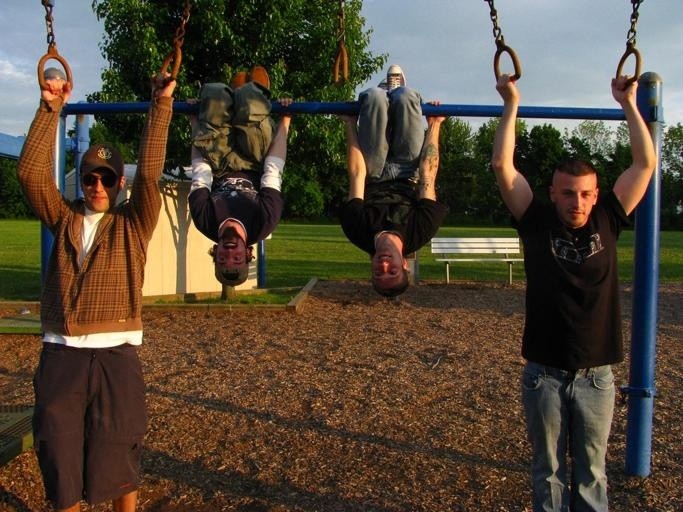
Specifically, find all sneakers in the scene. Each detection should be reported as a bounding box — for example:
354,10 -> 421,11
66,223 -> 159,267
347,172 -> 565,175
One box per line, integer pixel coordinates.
376,64 -> 406,91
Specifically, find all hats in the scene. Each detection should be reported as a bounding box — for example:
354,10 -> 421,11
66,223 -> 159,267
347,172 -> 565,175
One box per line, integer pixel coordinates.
80,142 -> 123,177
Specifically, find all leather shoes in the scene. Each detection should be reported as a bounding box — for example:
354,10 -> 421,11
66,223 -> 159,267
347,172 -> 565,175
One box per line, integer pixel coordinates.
229,65 -> 271,89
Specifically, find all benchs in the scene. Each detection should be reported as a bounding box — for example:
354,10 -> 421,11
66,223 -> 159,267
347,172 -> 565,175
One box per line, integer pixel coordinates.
429,237 -> 525,285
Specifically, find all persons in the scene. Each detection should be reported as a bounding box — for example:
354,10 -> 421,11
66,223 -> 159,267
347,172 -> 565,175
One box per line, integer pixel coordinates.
340,63 -> 445,297
186,66 -> 296,292
493,74 -> 658,511
15,71 -> 175,511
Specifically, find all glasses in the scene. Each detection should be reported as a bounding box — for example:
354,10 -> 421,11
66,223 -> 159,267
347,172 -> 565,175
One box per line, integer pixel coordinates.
82,174 -> 118,189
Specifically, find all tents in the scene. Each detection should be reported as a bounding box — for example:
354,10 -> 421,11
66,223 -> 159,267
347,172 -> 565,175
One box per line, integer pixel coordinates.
62,162 -> 260,297
